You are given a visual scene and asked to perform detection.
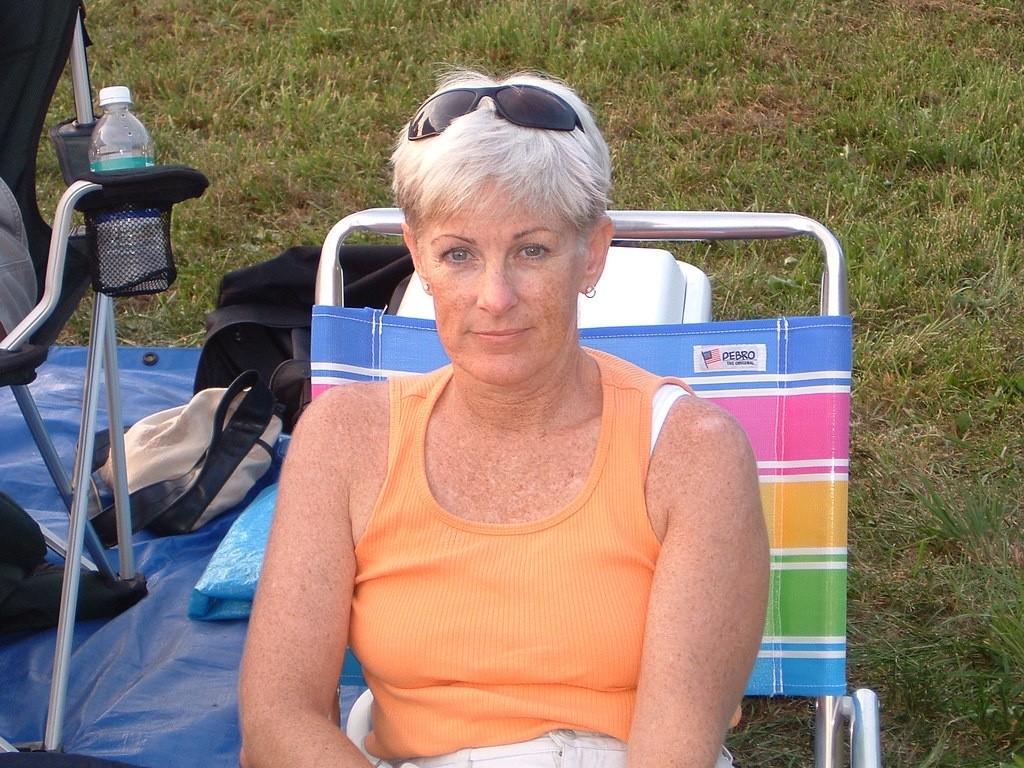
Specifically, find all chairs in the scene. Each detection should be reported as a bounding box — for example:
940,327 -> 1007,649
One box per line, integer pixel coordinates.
0,0 -> 209,757
309,206 -> 882,768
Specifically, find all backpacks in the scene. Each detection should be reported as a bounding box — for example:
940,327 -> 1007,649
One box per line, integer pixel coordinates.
75,370 -> 285,547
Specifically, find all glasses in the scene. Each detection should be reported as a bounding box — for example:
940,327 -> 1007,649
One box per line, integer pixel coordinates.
408,85 -> 586,139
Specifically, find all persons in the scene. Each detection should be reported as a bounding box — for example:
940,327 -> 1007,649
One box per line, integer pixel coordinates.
236,74 -> 771,767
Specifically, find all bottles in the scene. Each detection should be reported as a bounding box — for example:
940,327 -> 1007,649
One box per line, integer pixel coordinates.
87,85 -> 169,291
